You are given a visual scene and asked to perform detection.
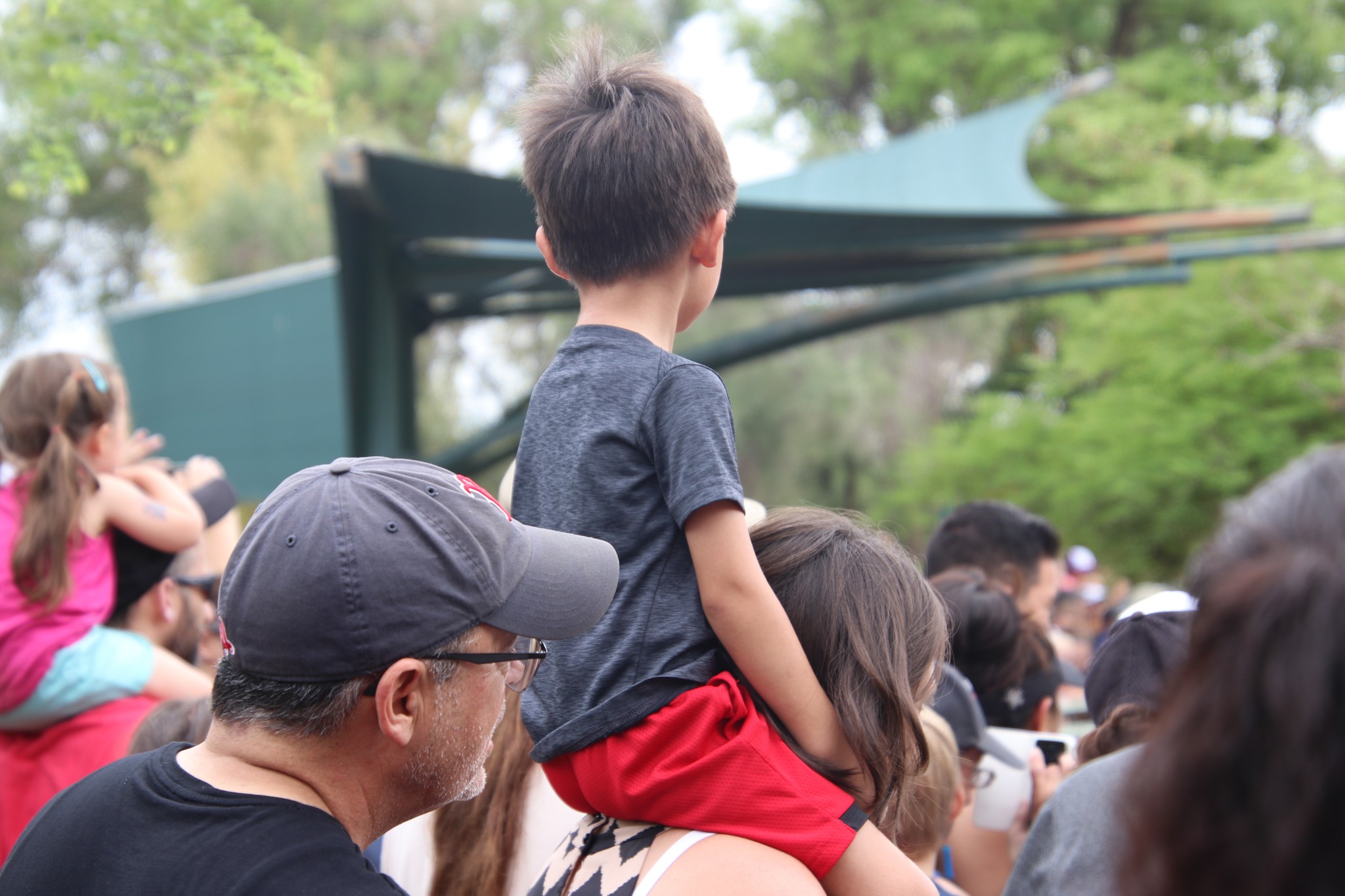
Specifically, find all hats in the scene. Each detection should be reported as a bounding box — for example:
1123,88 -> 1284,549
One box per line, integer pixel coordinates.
109,475 -> 235,612
218,456 -> 619,681
927,664 -> 1024,773
1083,590 -> 1200,729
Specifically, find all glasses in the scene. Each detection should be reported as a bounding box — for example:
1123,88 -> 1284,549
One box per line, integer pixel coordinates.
364,638 -> 548,698
166,574 -> 218,603
957,756 -> 993,787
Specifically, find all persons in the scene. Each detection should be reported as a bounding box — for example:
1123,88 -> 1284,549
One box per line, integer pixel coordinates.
923,499 -> 1127,742
530,506 -> 952,896
927,662 -> 1074,896
1077,591 -> 1199,767
511,53 -> 940,896
890,704 -> 966,896
0,509 -> 216,869
0,352 -> 214,731
1003,447 -> 1345,896
378,685 -> 587,896
0,455 -> 621,896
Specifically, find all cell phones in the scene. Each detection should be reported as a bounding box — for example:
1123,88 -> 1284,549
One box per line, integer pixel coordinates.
1036,739 -> 1066,767
